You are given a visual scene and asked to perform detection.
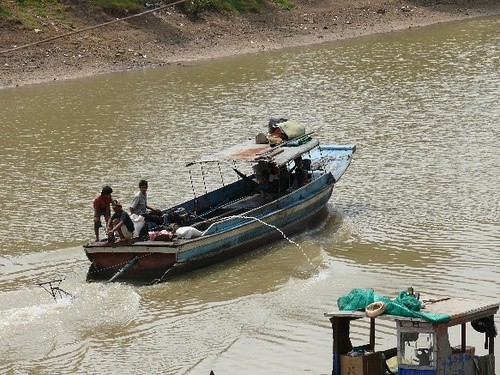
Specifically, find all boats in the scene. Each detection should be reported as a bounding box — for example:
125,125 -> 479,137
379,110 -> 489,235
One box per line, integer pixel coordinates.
82,116 -> 357,284
323,287 -> 499,375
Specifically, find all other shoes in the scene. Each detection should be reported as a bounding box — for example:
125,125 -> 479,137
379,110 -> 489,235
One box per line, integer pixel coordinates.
115,238 -> 125,243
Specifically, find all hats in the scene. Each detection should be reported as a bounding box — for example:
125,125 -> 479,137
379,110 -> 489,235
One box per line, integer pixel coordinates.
112,200 -> 122,208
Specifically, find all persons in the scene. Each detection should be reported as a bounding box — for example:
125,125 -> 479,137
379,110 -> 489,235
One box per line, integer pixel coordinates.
252,156 -> 308,202
93,186 -> 115,242
106,200 -> 134,244
130,180 -> 165,226
